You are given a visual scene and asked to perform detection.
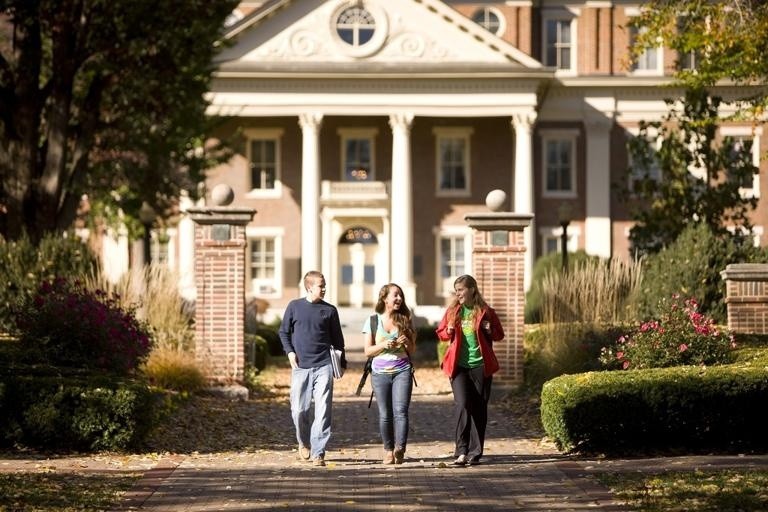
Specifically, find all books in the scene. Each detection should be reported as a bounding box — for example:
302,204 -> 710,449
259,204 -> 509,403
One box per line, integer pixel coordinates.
329,346 -> 344,379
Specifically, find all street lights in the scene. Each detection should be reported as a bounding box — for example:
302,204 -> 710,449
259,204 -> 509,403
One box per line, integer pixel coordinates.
555,200 -> 573,273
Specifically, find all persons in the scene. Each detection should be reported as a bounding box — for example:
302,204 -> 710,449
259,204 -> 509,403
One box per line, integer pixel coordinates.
432,273 -> 505,466
278,270 -> 348,466
360,281 -> 420,464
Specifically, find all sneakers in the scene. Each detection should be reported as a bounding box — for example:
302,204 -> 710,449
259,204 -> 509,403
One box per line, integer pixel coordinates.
383,450 -> 395,464
393,447 -> 405,465
454,454 -> 482,466
312,455 -> 326,466
301,446 -> 311,460
